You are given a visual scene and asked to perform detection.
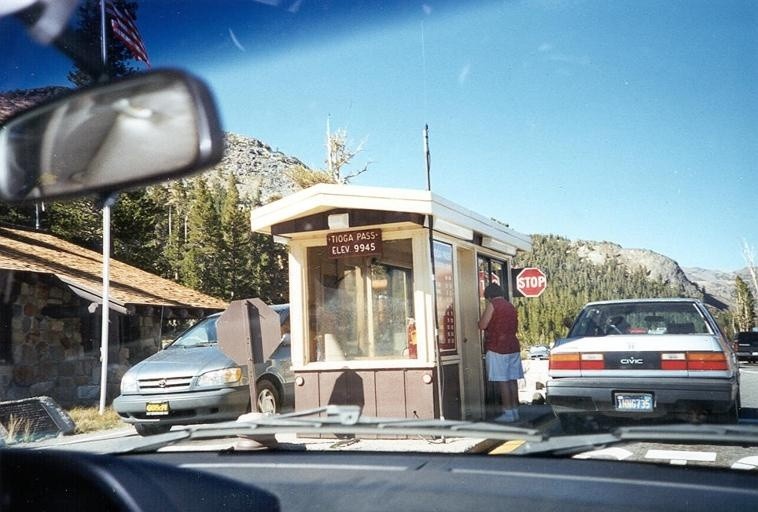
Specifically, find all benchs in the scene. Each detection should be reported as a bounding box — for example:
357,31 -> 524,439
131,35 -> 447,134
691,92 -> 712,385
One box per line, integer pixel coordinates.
603,316 -> 697,334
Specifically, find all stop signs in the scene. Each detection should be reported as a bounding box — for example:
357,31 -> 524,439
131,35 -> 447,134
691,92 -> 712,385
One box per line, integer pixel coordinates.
476,271 -> 500,300
515,267 -> 548,299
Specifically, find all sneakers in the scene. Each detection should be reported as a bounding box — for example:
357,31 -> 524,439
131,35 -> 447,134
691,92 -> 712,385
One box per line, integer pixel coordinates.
493,412 -> 519,423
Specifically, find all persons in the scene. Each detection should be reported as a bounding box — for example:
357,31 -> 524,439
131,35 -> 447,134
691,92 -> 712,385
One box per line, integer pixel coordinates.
477,283 -> 525,425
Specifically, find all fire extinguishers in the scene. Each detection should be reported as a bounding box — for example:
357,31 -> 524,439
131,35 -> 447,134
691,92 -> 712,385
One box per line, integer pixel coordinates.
406,318 -> 417,359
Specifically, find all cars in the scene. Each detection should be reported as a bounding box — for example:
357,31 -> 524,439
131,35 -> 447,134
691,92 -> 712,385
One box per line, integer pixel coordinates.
545,293 -> 747,441
528,344 -> 550,361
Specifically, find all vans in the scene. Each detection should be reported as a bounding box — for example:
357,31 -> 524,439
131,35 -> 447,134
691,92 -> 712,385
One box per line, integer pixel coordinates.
731,329 -> 758,366
110,301 -> 292,438
